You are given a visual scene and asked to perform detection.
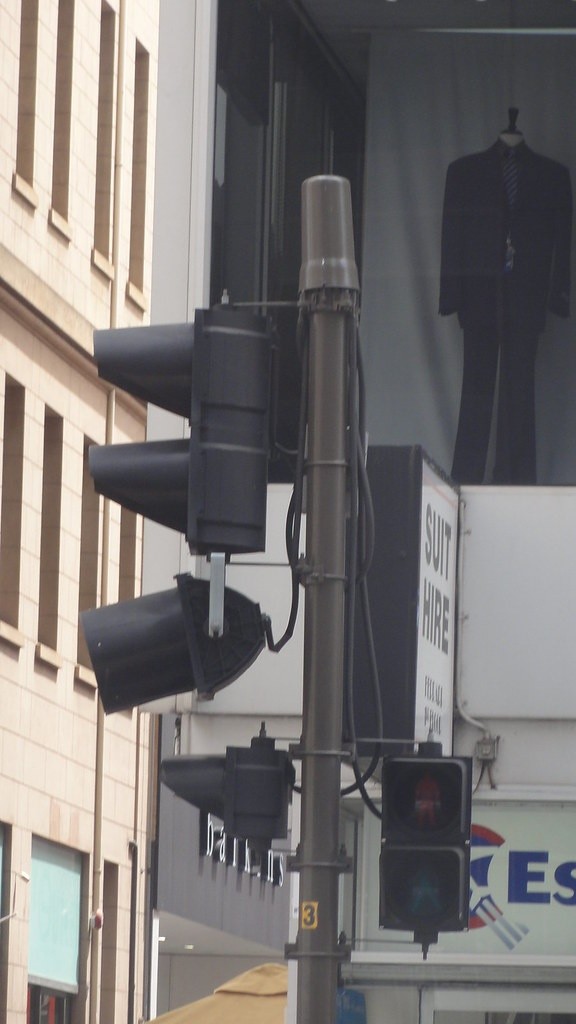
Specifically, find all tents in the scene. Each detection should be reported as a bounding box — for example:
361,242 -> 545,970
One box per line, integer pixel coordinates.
143,962 -> 291,1024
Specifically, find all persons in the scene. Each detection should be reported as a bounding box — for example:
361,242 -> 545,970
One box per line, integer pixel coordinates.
438,129 -> 572,487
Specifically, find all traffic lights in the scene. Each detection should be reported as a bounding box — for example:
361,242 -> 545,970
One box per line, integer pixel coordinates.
75,305 -> 279,716
377,761 -> 474,932
157,746 -> 293,839
